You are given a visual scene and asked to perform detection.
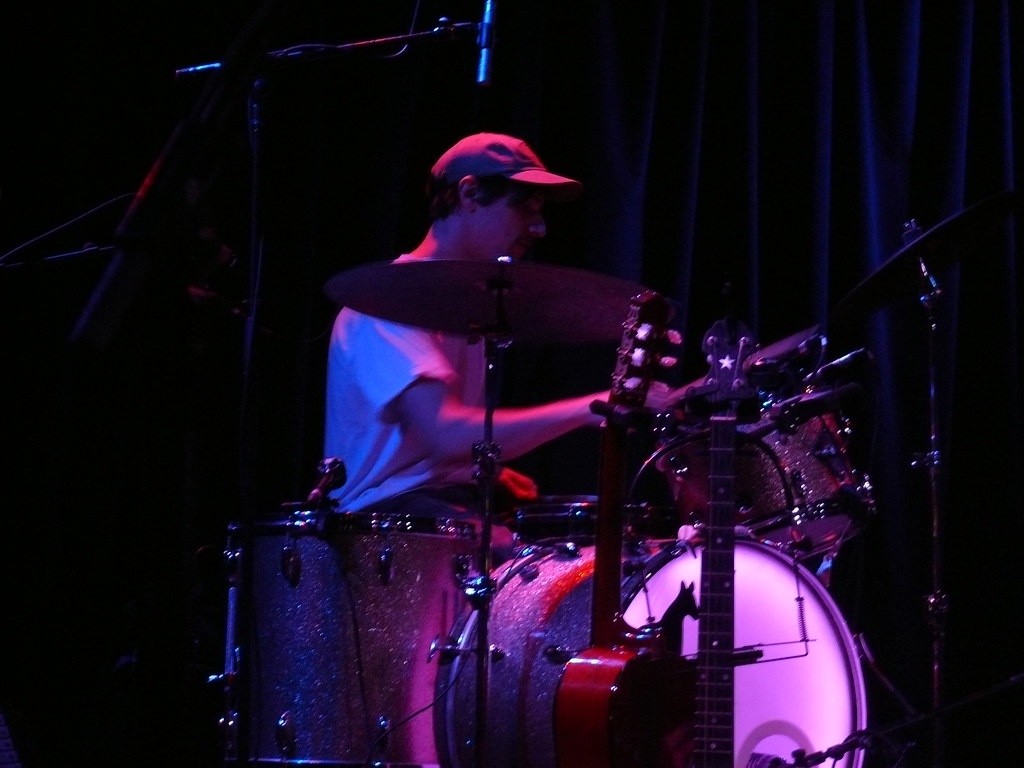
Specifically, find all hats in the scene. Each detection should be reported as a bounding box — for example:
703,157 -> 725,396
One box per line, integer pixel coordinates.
429,131 -> 581,205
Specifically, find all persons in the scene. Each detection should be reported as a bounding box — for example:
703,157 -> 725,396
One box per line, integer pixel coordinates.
323,132 -> 671,522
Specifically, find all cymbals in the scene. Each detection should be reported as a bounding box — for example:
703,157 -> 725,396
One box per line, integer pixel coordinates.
320,259 -> 672,348
845,208 -> 971,298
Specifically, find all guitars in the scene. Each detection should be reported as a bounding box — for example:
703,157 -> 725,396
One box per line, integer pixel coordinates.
684,319 -> 748,768
552,289 -> 667,768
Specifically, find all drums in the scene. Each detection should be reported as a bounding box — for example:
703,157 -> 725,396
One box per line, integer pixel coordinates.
663,325 -> 821,408
242,506 -> 475,768
506,495 -> 651,536
658,389 -> 868,554
433,522 -> 868,768
